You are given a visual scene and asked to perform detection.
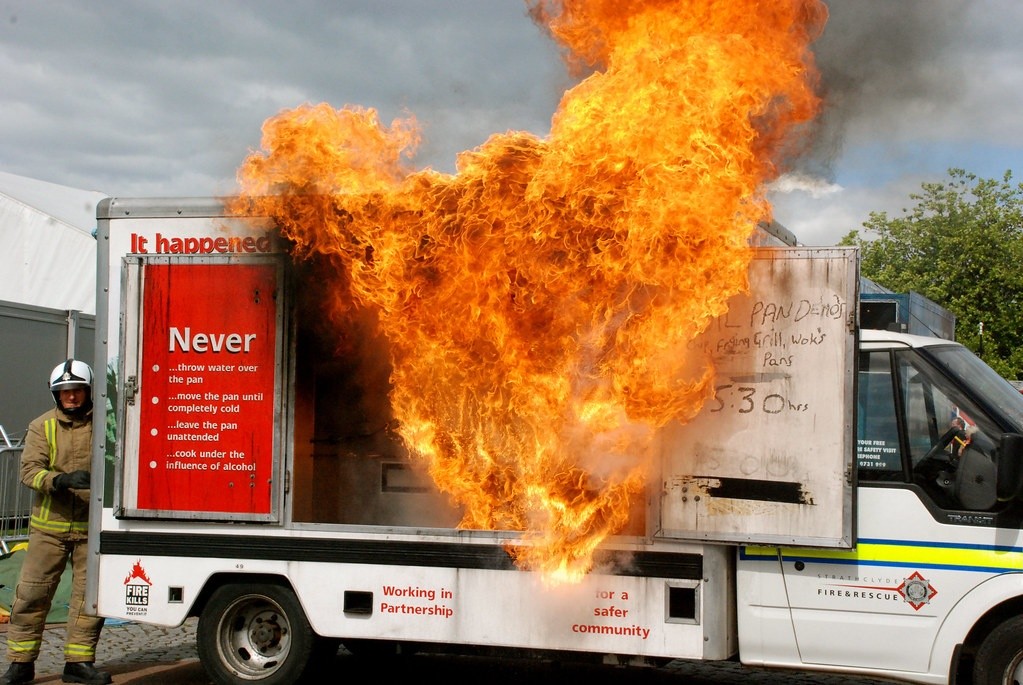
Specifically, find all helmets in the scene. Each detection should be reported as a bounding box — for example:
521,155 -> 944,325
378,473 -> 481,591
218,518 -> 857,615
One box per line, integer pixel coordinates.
48,359 -> 94,406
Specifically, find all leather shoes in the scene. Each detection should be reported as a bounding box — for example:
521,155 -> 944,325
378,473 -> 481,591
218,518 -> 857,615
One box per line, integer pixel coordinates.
62,661 -> 112,685
1,662 -> 36,685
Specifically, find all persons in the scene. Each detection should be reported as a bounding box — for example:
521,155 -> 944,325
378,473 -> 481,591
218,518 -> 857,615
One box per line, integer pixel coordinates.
0,359 -> 114,685
951,405 -> 978,455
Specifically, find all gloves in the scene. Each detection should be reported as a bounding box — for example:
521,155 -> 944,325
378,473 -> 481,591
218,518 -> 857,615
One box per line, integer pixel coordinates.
55,471 -> 92,491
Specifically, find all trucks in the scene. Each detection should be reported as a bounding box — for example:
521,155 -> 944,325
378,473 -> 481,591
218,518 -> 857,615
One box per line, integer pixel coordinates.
82,193 -> 1023,685
